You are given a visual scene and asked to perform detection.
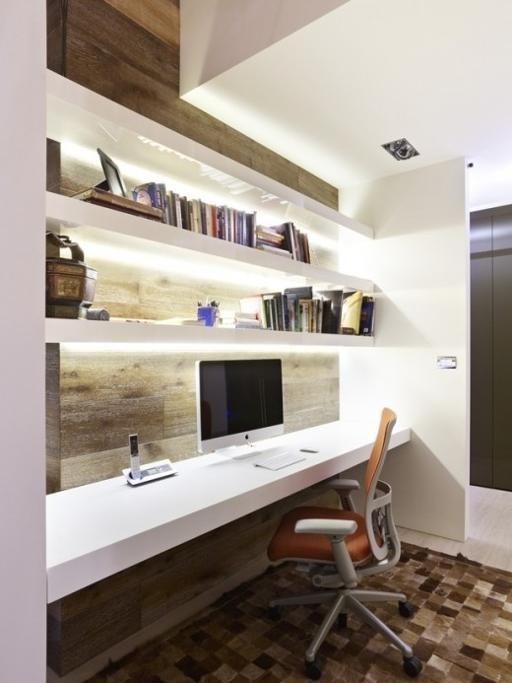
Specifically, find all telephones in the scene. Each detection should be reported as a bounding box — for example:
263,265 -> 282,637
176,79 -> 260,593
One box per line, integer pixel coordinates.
122,433 -> 178,487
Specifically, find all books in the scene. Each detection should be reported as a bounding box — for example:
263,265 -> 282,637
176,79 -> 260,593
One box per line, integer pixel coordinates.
130,182 -> 310,263
217,286 -> 376,337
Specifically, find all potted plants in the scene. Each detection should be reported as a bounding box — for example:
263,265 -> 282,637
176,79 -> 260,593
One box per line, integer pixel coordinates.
197,297 -> 224,327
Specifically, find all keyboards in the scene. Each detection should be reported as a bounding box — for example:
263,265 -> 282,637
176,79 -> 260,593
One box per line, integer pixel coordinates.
255,451 -> 305,472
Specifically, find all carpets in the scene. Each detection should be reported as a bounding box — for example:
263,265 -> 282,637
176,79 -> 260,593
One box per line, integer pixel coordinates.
82,541 -> 512,683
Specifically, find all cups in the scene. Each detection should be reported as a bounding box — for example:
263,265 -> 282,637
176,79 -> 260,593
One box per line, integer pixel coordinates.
198,307 -> 218,326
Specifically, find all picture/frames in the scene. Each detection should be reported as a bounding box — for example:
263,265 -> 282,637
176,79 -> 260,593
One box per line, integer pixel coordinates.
95,147 -> 128,199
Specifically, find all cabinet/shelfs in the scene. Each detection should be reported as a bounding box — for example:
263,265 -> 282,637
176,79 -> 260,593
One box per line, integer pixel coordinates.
45,71 -> 413,606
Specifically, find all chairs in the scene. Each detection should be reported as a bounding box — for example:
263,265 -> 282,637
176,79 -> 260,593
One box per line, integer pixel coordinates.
266,407 -> 423,680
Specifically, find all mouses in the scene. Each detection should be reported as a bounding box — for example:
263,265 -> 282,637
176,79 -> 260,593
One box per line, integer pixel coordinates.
299,449 -> 320,455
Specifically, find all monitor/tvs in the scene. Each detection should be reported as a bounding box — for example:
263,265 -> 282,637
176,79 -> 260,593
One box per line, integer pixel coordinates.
195,358 -> 285,461
